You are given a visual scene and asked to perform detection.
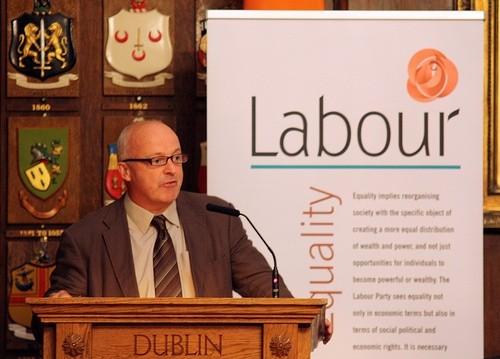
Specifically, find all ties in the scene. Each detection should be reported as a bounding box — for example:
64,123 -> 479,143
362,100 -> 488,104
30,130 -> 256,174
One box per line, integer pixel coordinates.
152,216 -> 182,297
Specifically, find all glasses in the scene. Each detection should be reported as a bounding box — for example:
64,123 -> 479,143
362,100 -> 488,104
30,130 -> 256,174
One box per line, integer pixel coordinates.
121,153 -> 190,166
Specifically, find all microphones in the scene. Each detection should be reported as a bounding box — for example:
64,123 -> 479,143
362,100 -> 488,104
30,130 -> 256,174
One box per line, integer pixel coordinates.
206,203 -> 280,298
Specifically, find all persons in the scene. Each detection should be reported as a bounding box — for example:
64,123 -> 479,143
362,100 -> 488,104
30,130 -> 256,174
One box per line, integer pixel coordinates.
32,119 -> 333,349
